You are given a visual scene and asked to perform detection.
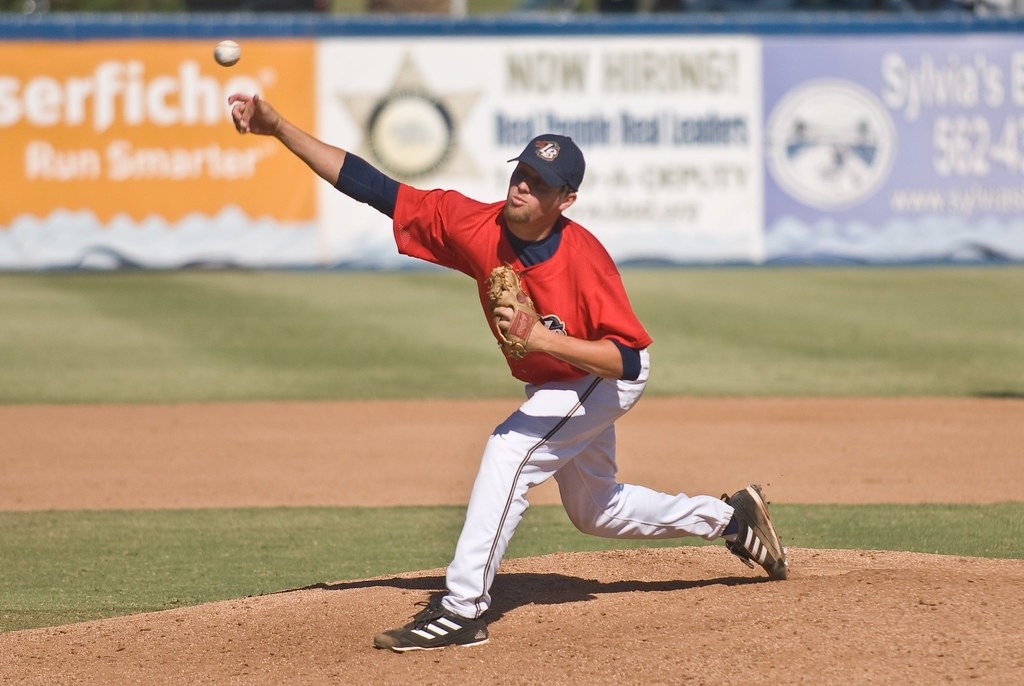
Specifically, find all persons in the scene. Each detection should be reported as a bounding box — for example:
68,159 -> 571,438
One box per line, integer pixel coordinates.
227,91 -> 789,652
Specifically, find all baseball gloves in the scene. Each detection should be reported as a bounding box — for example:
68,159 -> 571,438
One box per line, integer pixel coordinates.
485,268 -> 539,362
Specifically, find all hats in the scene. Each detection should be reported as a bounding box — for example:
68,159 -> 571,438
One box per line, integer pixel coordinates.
507,134 -> 585,192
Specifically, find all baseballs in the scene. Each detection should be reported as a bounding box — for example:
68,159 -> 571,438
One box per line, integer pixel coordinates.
214,39 -> 239,65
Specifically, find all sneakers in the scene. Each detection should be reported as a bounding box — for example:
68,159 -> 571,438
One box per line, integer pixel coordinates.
719,485 -> 788,580
374,599 -> 490,652
